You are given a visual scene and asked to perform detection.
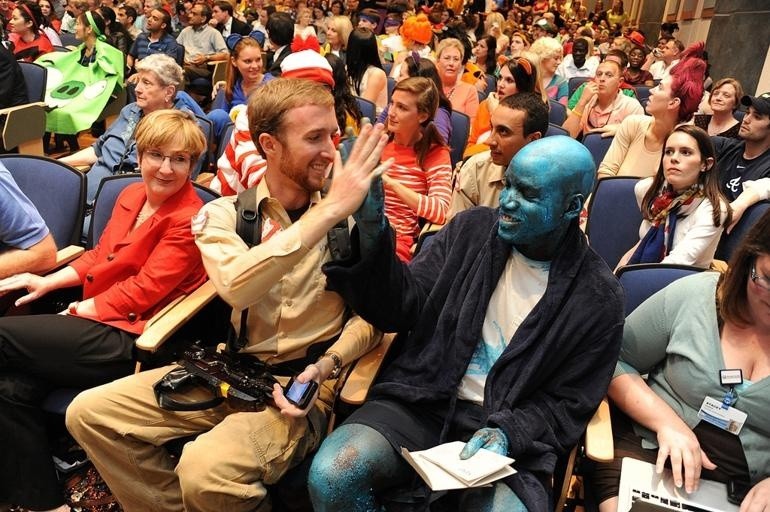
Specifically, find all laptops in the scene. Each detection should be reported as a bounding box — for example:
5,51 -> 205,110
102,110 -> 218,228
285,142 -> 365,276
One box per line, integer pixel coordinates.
616,455 -> 741,512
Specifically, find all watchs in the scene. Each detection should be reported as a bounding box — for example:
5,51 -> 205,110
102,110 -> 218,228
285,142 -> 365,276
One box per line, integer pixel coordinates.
323,354 -> 345,381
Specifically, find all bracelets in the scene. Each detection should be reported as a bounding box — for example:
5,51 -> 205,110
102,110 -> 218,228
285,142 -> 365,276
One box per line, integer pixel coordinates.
66,301 -> 78,314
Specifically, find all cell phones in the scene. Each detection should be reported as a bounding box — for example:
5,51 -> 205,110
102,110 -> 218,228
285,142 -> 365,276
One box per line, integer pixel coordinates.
729,480 -> 754,503
282,374 -> 317,412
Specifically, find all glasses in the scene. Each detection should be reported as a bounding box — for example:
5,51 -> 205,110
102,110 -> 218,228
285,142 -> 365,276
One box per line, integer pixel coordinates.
145,150 -> 193,165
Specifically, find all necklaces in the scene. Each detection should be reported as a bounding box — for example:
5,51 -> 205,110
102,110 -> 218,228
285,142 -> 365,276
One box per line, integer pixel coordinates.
134,209 -> 155,225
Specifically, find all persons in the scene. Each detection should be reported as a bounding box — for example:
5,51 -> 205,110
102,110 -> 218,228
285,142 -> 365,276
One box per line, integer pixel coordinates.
306,134 -> 628,511
1,0 -> 769,279
64,77 -> 396,512
0,107 -> 208,512
594,206 -> 770,511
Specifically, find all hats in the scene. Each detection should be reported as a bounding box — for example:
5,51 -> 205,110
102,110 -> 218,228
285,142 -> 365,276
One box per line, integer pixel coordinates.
400,15 -> 433,44
534,19 -> 551,32
625,30 -> 647,47
279,36 -> 334,88
741,93 -> 770,115
513,31 -> 533,47
